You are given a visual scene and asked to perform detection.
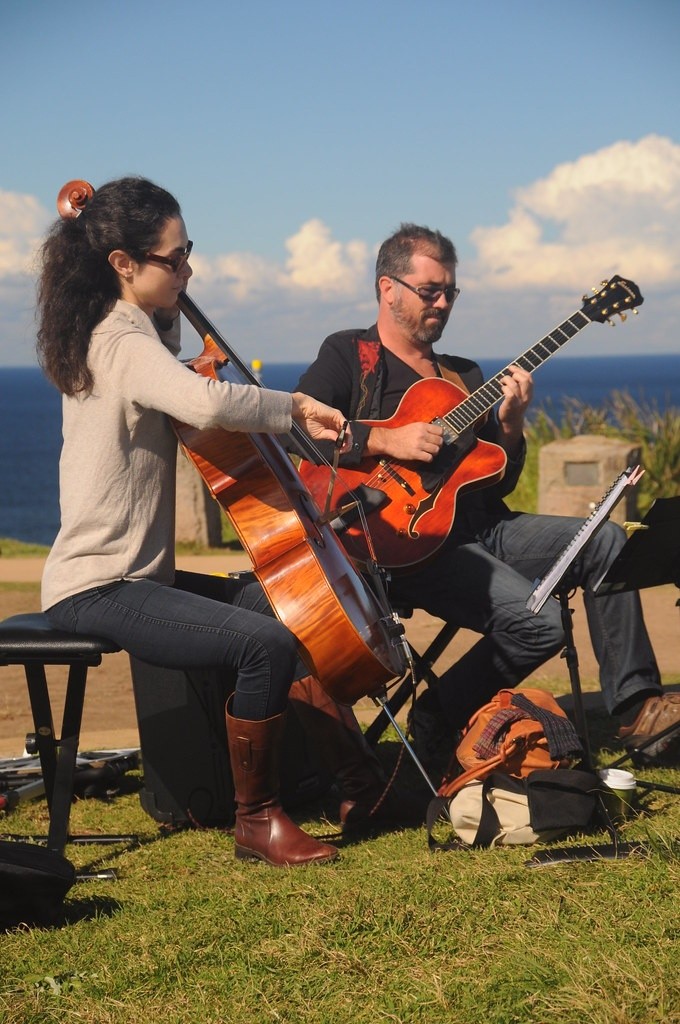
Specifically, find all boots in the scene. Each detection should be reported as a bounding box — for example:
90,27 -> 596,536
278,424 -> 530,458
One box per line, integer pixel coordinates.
225,691 -> 338,868
288,675 -> 423,837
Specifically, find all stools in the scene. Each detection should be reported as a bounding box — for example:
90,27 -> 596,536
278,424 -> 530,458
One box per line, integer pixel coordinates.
0,642 -> 139,882
364,598 -> 460,748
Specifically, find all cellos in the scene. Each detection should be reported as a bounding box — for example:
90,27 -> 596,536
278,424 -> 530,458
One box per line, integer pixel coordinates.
54,181 -> 452,825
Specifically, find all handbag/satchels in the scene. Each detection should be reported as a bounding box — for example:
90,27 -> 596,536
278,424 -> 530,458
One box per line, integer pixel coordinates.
438,688 -> 581,798
428,769 -> 602,852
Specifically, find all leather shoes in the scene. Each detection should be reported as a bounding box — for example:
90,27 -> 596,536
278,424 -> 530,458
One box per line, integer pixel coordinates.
614,692 -> 680,769
407,690 -> 458,770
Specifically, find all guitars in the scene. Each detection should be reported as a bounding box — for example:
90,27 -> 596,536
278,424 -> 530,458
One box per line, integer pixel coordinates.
298,271 -> 644,579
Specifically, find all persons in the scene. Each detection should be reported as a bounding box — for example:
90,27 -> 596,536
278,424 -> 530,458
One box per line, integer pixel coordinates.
274,225 -> 680,781
35,176 -> 428,868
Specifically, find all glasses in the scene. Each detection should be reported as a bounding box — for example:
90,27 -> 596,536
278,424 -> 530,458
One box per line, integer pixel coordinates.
390,275 -> 460,302
136,240 -> 193,272
